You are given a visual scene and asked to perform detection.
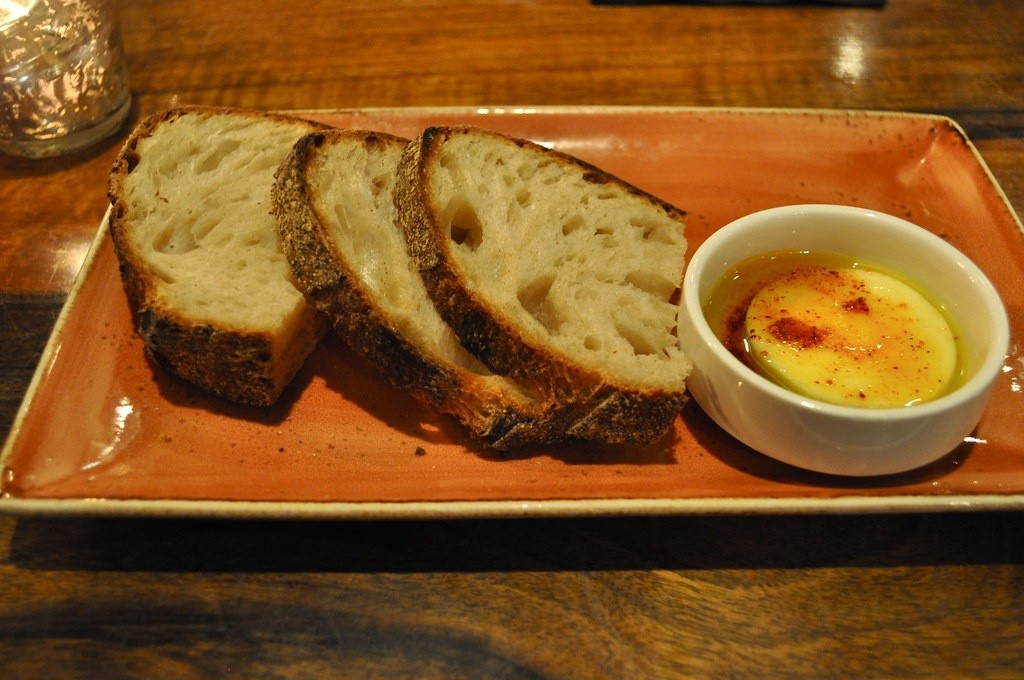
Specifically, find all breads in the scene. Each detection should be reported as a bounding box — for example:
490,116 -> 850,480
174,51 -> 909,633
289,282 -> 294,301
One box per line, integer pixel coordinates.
107,104 -> 693,446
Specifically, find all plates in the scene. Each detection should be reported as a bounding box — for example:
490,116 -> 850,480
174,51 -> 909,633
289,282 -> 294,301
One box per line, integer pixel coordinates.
0,106 -> 1024,520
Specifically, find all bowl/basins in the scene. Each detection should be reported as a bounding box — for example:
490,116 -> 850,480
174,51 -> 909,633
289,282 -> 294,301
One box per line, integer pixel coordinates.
677,203 -> 1010,477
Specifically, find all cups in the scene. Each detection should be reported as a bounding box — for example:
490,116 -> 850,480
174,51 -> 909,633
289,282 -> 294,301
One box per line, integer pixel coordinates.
0,0 -> 134,160
